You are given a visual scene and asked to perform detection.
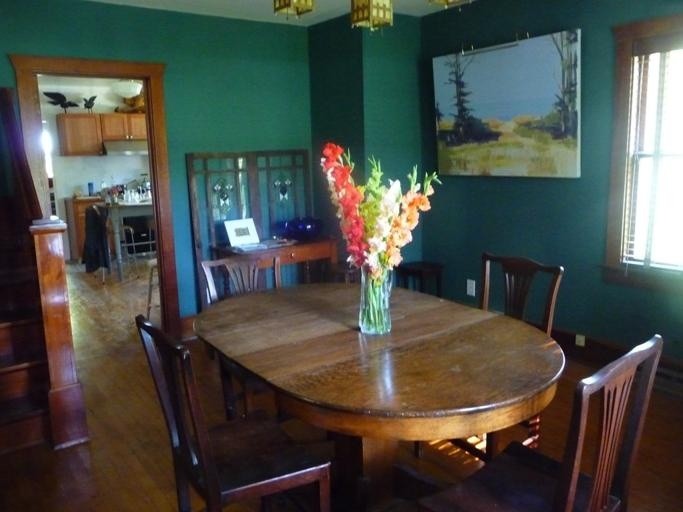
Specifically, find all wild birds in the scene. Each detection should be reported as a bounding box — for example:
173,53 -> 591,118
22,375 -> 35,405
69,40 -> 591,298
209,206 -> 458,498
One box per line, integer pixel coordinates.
42,91 -> 79,114
83,94 -> 98,112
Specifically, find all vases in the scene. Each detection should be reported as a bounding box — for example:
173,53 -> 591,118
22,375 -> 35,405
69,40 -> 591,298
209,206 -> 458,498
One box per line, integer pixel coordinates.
358,261 -> 394,335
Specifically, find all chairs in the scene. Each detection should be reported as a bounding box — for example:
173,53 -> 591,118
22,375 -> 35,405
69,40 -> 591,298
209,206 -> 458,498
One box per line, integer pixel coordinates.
387,328 -> 664,511
414,251 -> 565,468
134,313 -> 332,512
199,254 -> 282,422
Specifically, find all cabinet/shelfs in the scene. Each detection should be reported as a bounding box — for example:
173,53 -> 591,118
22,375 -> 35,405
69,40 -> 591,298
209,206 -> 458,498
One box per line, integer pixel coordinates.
55,113 -> 105,157
63,196 -> 114,266
99,112 -> 147,142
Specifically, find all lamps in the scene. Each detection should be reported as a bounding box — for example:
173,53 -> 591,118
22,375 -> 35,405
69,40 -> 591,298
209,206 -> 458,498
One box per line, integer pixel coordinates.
272,0 -> 472,36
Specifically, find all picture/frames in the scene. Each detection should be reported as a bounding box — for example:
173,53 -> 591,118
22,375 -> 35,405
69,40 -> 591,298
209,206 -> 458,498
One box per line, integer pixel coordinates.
223,217 -> 260,248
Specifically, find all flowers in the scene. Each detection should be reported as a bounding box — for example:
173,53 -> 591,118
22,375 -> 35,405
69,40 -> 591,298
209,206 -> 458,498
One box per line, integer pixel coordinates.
319,142 -> 445,332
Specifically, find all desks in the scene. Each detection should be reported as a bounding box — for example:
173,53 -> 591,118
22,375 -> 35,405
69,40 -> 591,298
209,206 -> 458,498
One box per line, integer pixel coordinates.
95,202 -> 157,285
190,281 -> 566,511
210,236 -> 341,297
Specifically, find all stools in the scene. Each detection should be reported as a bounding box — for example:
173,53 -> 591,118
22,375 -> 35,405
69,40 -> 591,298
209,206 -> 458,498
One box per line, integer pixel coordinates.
394,260 -> 445,297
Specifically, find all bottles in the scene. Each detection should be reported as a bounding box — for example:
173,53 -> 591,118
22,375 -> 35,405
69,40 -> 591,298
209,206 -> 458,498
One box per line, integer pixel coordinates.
103,172 -> 150,206
